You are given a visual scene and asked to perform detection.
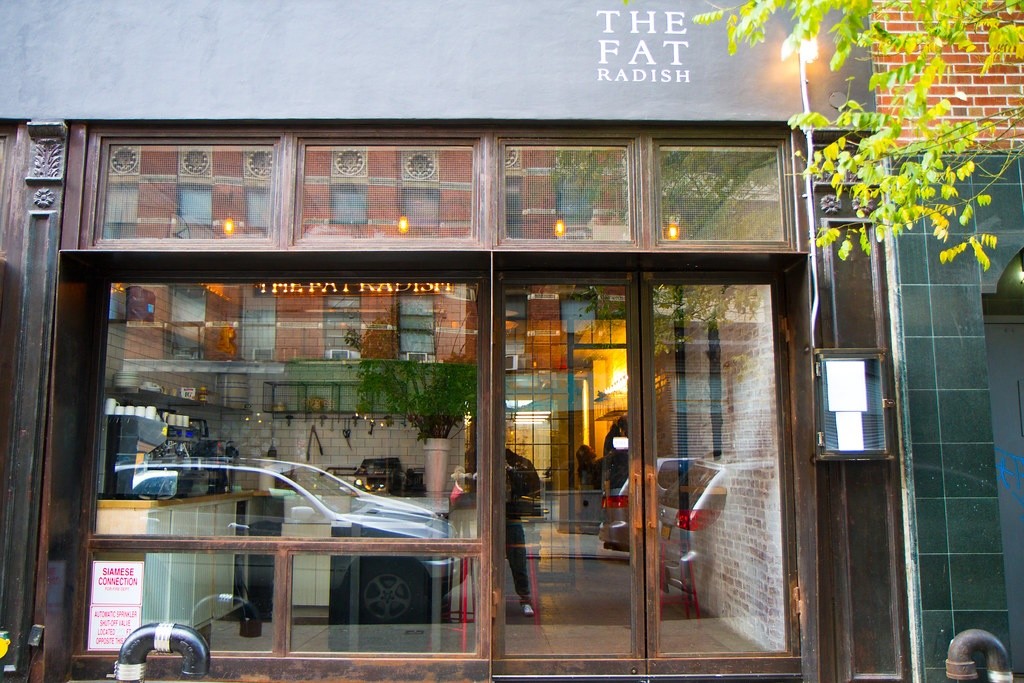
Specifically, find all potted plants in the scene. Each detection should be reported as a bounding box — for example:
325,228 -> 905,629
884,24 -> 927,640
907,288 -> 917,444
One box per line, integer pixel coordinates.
348,291 -> 478,492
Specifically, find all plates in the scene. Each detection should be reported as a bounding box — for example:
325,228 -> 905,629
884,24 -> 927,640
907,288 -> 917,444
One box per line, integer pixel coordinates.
146,387 -> 160,393
115,372 -> 138,388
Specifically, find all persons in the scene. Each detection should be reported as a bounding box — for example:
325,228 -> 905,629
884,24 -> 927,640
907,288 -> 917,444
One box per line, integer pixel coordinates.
576,445 -> 616,571
603,415 -> 628,486
473,448 -> 540,617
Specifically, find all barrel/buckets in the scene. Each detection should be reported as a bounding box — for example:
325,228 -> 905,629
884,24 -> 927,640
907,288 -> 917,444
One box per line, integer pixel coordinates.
215,373 -> 249,409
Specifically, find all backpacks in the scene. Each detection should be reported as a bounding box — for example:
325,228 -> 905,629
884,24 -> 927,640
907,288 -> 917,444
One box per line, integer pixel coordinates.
506,447 -> 546,523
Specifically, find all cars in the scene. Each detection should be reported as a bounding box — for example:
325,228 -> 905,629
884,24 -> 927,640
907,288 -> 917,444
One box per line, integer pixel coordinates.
108,455 -> 468,623
595,455 -> 703,553
661,459 -> 775,597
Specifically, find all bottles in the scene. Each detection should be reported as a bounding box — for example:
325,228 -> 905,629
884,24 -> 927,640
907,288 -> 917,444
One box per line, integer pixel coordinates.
198,387 -> 208,402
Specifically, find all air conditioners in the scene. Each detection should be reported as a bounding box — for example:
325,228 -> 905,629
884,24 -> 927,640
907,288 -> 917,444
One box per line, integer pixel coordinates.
253,348 -> 274,360
330,350 -> 350,360
506,355 -> 518,371
406,353 -> 428,363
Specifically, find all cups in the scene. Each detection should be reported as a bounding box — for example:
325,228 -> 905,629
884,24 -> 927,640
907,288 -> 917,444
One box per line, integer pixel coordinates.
104,398 -> 160,422
143,382 -> 158,387
162,412 -> 189,427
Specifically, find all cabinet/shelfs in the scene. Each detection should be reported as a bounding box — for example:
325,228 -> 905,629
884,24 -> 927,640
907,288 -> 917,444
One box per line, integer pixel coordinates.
89,500 -> 249,631
280,523 -> 331,607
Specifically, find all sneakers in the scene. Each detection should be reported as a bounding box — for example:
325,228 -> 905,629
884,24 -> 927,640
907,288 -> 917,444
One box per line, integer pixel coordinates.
521,604 -> 534,616
